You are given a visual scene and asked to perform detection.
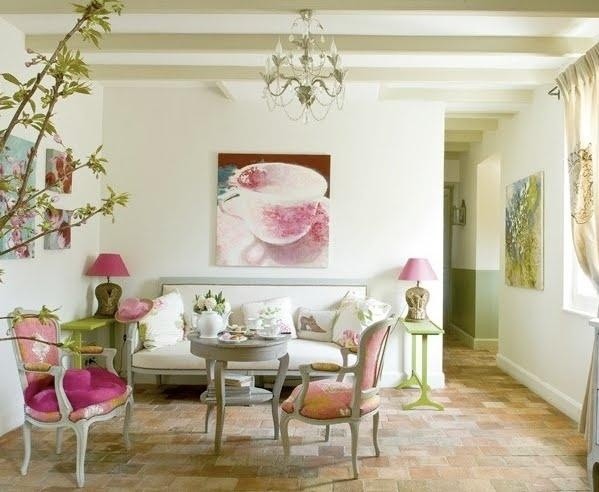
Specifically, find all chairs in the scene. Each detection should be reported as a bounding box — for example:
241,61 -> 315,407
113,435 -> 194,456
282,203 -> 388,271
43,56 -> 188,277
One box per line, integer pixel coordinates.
280,318 -> 395,479
10,314 -> 134,488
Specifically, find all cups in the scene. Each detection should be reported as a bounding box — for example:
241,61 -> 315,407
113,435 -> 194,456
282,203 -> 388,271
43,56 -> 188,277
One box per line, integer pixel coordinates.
218,163 -> 329,245
263,324 -> 280,335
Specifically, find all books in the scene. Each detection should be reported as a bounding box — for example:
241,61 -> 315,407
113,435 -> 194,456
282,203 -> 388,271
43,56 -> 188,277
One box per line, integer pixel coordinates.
207,373 -> 252,400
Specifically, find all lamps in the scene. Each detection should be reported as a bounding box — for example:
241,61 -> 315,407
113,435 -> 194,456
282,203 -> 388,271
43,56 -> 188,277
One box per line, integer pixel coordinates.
399,258 -> 437,323
86,254 -> 130,319
259,9 -> 350,121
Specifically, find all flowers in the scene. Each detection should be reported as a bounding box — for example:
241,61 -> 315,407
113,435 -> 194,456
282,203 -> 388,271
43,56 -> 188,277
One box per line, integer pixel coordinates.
193,290 -> 225,316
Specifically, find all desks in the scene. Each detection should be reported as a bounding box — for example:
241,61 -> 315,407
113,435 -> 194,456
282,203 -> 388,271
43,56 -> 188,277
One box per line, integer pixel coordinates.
188,332 -> 289,456
396,319 -> 445,410
61,317 -> 116,369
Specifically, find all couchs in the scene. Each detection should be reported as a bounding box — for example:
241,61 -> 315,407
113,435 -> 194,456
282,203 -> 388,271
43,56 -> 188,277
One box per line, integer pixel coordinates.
126,277 -> 371,404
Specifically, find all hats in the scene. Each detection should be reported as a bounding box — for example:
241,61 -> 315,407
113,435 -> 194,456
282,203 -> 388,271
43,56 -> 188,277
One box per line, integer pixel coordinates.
115,298 -> 153,324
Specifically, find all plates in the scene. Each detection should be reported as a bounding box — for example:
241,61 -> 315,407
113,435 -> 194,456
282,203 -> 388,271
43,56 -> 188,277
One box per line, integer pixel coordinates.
219,336 -> 248,343
259,334 -> 287,340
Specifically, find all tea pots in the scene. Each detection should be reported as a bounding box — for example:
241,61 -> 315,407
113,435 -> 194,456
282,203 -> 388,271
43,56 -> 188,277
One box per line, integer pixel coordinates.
191,306 -> 234,339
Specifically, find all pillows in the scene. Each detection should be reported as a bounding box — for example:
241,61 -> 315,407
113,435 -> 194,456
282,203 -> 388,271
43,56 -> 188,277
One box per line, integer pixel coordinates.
138,288 -> 186,351
297,308 -> 340,342
332,291 -> 392,353
242,297 -> 297,339
